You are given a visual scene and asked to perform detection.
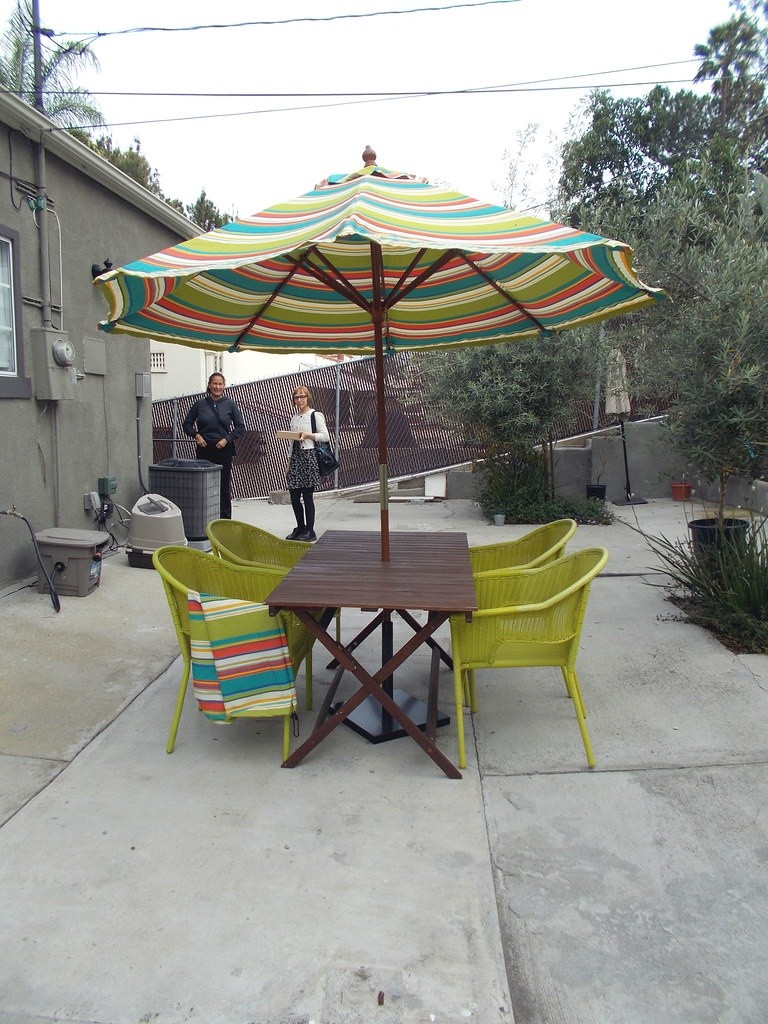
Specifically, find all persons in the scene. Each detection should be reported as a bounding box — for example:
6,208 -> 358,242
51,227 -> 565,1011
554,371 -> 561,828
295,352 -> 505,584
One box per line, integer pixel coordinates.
183,372 -> 246,519
286,386 -> 330,541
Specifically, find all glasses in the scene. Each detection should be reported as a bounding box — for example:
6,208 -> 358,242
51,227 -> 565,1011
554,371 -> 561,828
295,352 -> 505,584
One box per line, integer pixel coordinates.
294,395 -> 307,400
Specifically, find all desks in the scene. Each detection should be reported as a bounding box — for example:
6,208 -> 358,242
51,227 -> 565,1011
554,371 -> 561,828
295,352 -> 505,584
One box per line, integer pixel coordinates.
263,530 -> 479,776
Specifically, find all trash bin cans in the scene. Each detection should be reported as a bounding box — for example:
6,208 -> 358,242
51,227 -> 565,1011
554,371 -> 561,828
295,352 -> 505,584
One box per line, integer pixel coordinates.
147,458 -> 223,541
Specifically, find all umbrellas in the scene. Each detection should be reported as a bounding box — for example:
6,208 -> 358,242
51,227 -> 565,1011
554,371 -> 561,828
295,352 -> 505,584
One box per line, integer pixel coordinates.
97,143 -> 668,717
604,347 -> 632,501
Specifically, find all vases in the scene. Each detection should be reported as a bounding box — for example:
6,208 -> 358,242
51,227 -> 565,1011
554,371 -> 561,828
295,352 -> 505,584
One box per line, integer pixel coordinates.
586,485 -> 606,508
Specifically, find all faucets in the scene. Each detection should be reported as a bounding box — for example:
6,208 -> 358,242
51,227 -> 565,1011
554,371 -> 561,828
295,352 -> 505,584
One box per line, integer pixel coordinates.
7,504 -> 23,519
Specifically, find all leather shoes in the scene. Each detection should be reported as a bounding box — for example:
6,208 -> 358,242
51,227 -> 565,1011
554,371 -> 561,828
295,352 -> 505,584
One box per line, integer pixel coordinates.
286,527 -> 308,539
297,528 -> 317,542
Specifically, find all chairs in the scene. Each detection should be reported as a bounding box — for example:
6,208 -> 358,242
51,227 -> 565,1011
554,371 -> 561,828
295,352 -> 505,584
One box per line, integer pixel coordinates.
150,519 -> 340,765
449,518 -> 609,774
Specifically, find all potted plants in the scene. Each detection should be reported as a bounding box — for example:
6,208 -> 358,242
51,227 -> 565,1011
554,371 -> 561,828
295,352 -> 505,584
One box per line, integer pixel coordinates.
671,461 -> 692,500
662,309 -> 768,581
494,505 -> 506,526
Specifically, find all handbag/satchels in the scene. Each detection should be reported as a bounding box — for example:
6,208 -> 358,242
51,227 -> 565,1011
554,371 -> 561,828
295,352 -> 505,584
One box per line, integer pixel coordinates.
231,439 -> 237,457
311,411 -> 340,477
